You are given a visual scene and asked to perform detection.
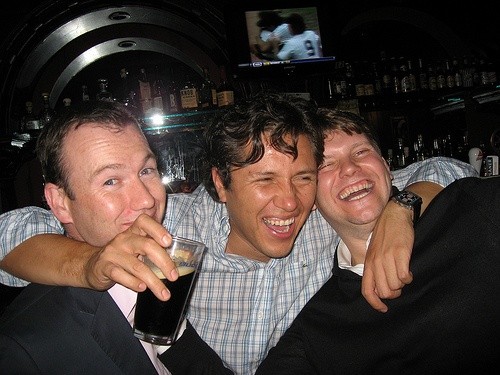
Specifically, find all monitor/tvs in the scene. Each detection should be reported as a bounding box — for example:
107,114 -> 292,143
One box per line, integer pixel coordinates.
245,7 -> 324,67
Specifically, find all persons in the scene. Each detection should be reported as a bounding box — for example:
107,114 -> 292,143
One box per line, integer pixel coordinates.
150,109 -> 500,375
250,11 -> 321,59
0,89 -> 478,375
0,97 -> 168,375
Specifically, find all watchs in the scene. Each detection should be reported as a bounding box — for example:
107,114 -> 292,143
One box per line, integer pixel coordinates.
386,189 -> 423,221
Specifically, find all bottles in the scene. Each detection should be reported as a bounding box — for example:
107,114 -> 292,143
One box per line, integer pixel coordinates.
327,56 -> 500,101
23,66 -> 236,137
395,134 -> 500,178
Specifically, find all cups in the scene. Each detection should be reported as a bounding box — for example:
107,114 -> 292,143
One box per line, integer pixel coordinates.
132,234 -> 205,346
